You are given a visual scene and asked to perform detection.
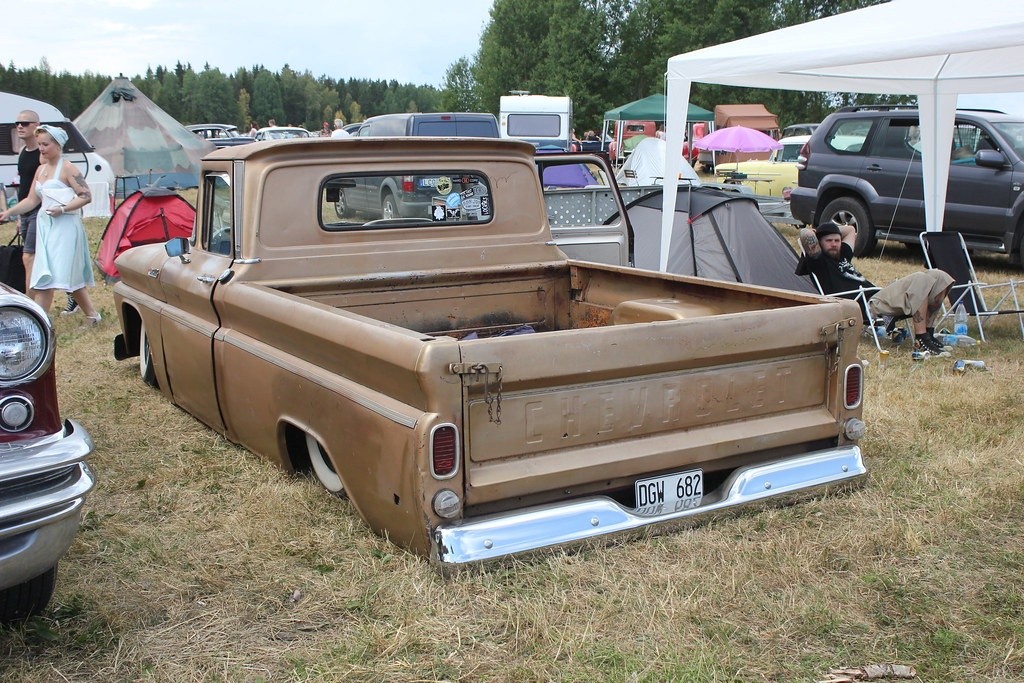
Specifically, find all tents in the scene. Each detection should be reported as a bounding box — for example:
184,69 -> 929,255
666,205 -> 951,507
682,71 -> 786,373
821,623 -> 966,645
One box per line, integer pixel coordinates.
71,73 -> 218,199
97,186 -> 219,286
603,92 -> 717,175
661,0 -> 1024,276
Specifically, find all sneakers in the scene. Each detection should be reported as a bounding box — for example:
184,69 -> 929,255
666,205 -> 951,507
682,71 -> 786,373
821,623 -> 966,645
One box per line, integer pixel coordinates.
60,294 -> 79,314
911,333 -> 950,359
926,328 -> 954,351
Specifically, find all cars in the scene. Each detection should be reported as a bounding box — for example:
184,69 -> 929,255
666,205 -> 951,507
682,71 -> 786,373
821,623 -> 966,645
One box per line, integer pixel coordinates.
0,281 -> 97,613
717,124 -> 866,199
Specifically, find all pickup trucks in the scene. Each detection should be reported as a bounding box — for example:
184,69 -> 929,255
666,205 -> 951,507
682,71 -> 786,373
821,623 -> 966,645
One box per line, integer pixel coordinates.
113,135 -> 868,580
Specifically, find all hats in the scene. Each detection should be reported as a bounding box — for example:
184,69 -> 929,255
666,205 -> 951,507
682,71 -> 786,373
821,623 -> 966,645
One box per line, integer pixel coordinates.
815,222 -> 842,242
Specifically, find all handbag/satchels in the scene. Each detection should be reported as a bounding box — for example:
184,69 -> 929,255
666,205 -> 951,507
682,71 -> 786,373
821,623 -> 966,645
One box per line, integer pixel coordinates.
0,230 -> 27,294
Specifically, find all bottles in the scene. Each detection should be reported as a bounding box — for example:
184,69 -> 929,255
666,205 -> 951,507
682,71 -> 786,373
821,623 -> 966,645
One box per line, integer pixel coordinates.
952,360 -> 966,376
933,332 -> 981,348
880,351 -> 889,361
962,360 -> 992,370
954,301 -> 968,336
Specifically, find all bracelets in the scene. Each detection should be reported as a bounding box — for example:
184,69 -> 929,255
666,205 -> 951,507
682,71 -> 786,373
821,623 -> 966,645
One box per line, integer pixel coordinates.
61,206 -> 65,215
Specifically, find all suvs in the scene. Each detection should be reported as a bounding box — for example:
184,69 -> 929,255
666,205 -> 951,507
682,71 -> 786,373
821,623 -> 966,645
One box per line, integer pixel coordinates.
791,104 -> 1024,267
184,122 -> 363,188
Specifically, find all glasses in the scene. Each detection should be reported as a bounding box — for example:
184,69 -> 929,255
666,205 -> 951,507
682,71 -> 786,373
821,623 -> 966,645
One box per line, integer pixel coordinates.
15,121 -> 37,127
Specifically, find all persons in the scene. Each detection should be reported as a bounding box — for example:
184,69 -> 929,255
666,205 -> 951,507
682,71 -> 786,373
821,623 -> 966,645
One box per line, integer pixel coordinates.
906,125 -> 923,154
654,123 -> 690,144
0,111 -> 103,330
795,222 -> 955,358
571,127 -> 612,142
196,119 -> 351,139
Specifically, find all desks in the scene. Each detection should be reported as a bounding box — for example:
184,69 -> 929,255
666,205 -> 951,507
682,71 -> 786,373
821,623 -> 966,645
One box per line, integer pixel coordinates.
725,178 -> 774,197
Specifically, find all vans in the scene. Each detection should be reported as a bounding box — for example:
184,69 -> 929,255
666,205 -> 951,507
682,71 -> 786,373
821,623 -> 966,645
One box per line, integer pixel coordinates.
610,121 -> 656,142
335,112 -> 502,219
0,92 -> 114,218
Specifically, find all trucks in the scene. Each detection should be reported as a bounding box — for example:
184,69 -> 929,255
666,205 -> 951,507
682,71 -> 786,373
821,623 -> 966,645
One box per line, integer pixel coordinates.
498,90 -> 574,151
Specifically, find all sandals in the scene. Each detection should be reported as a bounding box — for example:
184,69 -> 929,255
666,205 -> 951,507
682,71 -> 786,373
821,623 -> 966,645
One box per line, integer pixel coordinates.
80,313 -> 101,330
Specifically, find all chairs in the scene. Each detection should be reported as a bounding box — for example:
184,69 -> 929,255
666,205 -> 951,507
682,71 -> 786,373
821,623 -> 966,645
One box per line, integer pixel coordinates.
799,237 -> 915,356
919,231 -> 1024,344
951,137 -> 997,159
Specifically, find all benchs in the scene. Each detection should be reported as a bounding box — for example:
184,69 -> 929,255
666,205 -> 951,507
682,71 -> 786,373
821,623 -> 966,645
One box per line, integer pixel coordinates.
714,169 -> 737,184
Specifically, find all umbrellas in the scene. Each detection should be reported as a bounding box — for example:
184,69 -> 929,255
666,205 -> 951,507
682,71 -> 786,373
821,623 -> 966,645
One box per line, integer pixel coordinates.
695,126 -> 785,173
